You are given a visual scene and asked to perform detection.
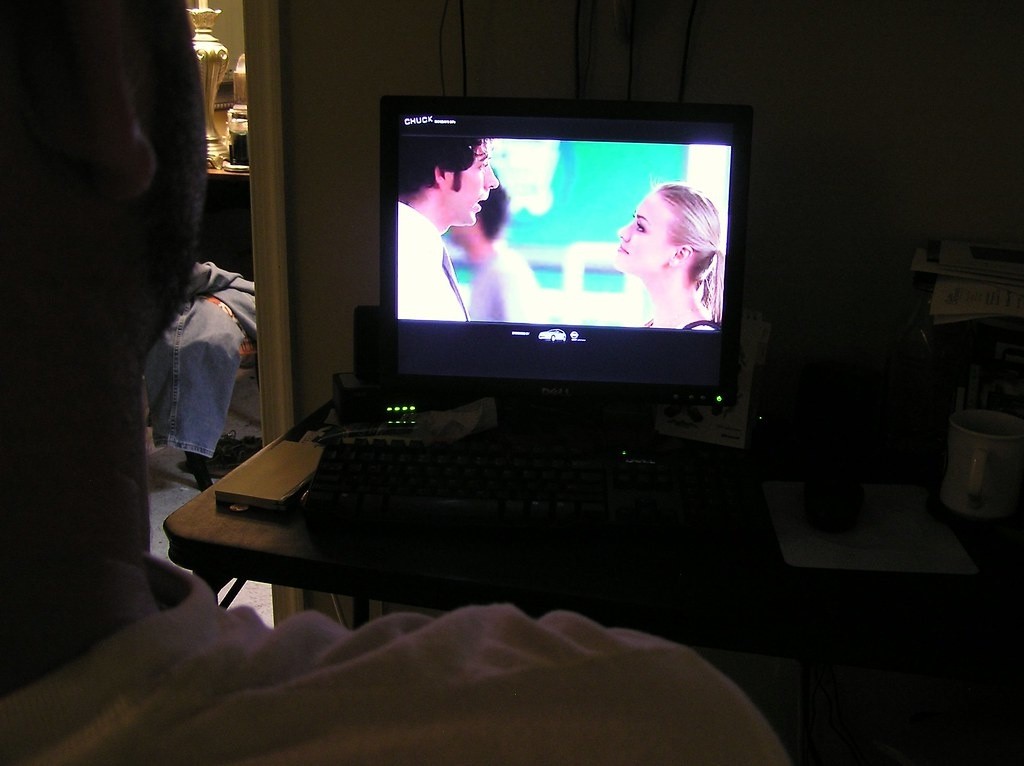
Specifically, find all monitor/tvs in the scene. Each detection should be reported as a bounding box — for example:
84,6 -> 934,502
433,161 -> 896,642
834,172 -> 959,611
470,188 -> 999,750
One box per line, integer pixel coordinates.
379,96 -> 754,431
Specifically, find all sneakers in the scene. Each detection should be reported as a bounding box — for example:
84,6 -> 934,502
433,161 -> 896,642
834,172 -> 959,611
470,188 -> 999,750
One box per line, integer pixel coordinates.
202,430 -> 262,475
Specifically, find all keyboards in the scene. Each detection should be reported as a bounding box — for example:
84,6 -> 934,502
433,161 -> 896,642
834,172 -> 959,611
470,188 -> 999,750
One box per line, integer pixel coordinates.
304,439 -> 708,553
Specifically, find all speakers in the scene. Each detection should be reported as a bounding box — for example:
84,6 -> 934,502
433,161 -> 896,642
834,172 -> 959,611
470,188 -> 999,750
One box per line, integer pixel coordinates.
352,306 -> 384,388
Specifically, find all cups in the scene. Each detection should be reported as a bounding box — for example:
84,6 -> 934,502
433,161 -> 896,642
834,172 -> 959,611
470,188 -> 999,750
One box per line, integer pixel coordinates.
938,409 -> 1024,517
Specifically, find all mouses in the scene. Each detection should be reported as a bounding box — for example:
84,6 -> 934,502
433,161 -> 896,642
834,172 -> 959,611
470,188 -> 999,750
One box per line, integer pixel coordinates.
803,465 -> 865,534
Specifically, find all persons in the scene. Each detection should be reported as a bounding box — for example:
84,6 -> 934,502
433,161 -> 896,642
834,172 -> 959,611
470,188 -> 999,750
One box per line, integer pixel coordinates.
614,183 -> 724,331
0,0 -> 790,766
396,136 -> 544,325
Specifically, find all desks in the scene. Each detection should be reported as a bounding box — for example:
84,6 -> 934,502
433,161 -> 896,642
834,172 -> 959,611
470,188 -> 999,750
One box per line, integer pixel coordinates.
163,400 -> 1023,765
